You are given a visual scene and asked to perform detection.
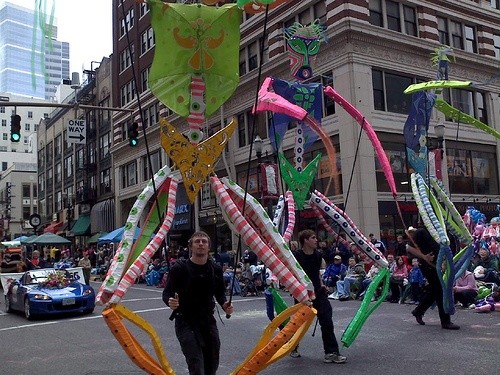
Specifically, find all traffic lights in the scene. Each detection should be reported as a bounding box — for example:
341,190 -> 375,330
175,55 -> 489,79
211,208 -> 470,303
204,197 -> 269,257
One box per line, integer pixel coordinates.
129,121 -> 139,147
10,114 -> 21,143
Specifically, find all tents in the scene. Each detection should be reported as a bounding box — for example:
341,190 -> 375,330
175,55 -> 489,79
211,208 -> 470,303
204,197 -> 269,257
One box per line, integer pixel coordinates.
1,231 -> 73,261
95,224 -> 156,259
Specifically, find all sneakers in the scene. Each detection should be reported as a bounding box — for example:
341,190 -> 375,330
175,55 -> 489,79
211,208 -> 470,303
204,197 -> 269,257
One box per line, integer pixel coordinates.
323,353 -> 347,364
289,352 -> 300,358
412,310 -> 425,325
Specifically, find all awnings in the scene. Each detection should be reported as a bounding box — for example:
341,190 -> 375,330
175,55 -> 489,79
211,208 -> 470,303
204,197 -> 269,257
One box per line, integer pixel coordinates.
32,217 -> 108,244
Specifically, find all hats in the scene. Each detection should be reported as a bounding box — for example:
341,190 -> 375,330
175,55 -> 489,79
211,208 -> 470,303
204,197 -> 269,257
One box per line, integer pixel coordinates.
334,255 -> 341,259
369,233 -> 374,237
408,226 -> 416,231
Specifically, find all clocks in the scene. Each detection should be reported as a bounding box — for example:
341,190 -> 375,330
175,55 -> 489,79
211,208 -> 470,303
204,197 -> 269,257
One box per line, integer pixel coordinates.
240,171 -> 259,194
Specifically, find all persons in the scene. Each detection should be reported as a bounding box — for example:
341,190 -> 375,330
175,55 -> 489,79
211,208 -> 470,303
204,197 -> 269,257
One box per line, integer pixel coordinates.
161,231 -> 232,375
0,225 -> 500,314
404,224 -> 461,329
321,255 -> 347,291
294,229 -> 347,363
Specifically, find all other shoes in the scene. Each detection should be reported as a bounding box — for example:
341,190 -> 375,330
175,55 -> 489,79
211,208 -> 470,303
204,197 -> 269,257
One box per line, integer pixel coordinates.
442,323 -> 460,330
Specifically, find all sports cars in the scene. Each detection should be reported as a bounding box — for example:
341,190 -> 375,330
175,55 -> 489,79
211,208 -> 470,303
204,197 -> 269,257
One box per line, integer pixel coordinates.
4,268 -> 96,320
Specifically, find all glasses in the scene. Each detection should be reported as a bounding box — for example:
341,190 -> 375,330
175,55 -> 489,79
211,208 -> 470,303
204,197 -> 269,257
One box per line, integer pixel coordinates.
408,231 -> 414,233
369,235 -> 373,237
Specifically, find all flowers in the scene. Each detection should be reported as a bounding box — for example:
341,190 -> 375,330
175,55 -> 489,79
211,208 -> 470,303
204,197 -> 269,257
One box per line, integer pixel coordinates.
36,269 -> 74,291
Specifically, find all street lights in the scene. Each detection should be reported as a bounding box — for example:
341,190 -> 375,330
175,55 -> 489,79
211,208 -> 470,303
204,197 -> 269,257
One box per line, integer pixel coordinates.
62,192 -> 77,230
254,134 -> 264,200
433,118 -> 446,157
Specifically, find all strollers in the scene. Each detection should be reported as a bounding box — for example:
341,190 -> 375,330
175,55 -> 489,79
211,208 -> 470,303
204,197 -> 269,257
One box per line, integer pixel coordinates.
240,265 -> 264,297
